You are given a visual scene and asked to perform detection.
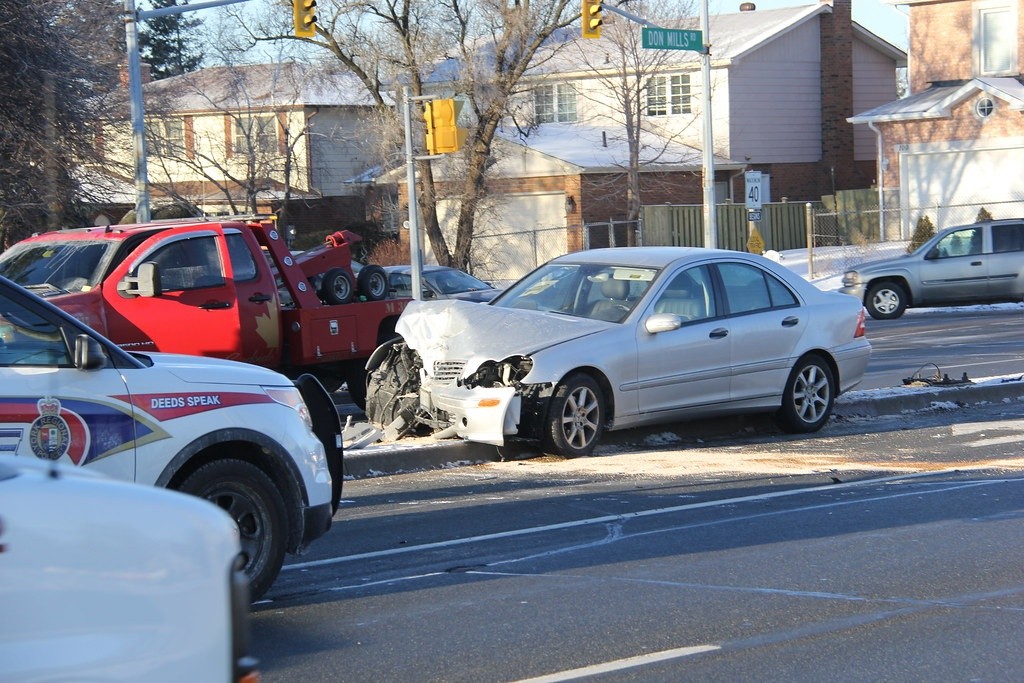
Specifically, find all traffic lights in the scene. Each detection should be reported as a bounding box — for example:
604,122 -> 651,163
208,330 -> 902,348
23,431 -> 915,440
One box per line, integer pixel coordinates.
294,0 -> 317,37
582,0 -> 603,38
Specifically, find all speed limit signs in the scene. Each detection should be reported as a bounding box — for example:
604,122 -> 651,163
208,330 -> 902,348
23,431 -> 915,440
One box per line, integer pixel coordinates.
746,172 -> 762,208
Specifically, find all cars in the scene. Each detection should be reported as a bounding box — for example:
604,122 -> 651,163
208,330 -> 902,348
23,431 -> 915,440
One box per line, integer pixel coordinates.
383,265 -> 503,304
0,453 -> 262,682
366,246 -> 871,457
838,218 -> 1024,320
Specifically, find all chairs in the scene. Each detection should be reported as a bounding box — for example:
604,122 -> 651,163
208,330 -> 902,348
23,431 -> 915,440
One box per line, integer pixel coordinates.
590,276 -> 634,321
656,273 -> 706,318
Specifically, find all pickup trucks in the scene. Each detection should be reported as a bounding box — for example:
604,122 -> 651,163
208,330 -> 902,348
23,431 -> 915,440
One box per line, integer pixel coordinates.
0,215 -> 415,412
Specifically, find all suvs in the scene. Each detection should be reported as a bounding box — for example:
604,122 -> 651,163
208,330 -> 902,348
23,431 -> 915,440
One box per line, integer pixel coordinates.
0,276 -> 343,602
